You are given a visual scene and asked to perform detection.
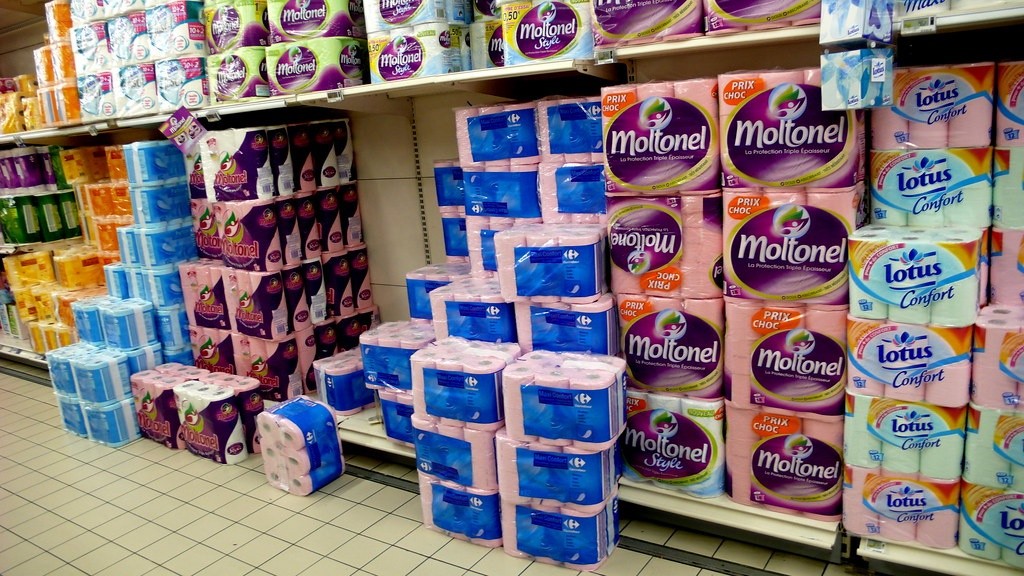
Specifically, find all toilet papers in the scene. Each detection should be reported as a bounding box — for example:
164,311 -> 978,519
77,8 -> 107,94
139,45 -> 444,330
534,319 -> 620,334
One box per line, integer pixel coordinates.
0,0 -> 1024,571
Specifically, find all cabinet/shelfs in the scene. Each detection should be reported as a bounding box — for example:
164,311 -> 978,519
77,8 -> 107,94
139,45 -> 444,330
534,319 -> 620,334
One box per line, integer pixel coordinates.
0,1 -> 1024,575
